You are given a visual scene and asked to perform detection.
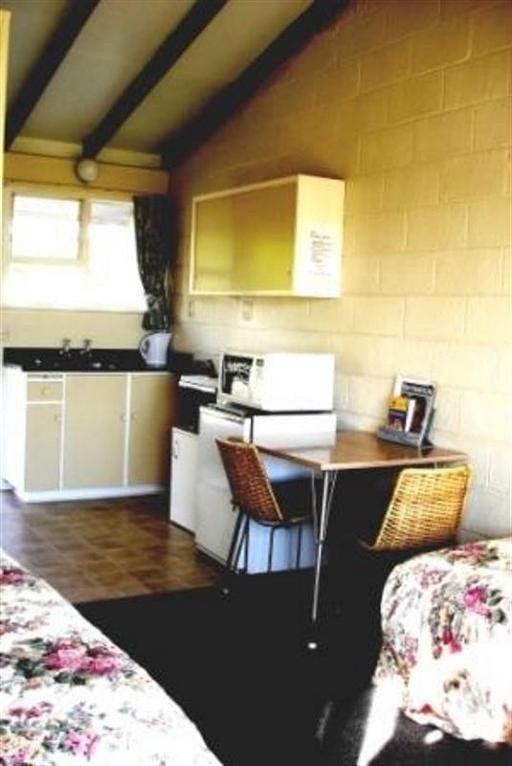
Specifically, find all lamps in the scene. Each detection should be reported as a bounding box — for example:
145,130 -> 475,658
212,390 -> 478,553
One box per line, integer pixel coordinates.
77,158 -> 100,181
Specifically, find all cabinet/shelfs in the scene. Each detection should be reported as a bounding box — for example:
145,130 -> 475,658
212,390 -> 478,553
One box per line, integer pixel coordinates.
60,363 -> 178,502
189,173 -> 345,300
5,367 -> 61,502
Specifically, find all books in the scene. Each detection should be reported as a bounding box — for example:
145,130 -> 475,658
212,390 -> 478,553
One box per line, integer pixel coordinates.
390,375 -> 437,439
385,394 -> 417,433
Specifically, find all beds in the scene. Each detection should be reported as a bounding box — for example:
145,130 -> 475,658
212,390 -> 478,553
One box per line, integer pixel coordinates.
376,536 -> 512,746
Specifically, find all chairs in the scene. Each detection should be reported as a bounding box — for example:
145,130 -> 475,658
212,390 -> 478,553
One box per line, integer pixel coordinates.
353,462 -> 472,627
215,437 -> 330,597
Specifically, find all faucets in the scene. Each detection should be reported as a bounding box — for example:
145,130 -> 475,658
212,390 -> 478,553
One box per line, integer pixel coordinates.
80,339 -> 92,356
58,339 -> 71,356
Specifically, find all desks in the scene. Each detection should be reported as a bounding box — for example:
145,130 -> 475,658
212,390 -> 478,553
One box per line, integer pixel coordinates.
255,429 -> 468,621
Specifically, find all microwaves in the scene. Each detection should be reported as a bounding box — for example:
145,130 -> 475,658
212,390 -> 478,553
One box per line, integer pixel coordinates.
217,349 -> 336,413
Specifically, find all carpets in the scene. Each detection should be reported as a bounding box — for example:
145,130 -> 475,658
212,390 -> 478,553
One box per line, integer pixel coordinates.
72,554 -> 512,764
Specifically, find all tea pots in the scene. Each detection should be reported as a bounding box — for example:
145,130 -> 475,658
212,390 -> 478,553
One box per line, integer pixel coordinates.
140,333 -> 174,366
